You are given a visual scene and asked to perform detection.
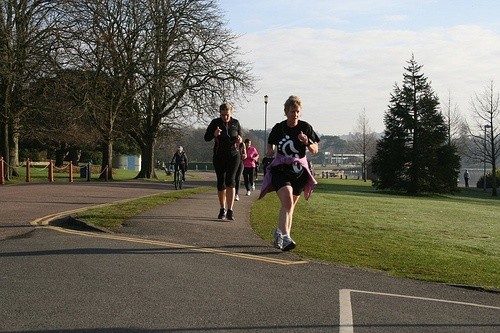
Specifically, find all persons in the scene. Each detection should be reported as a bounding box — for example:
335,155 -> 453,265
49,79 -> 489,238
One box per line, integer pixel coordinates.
171,145 -> 188,185
257,95 -> 320,252
464,169 -> 470,187
204,103 -> 242,220
234,139 -> 275,201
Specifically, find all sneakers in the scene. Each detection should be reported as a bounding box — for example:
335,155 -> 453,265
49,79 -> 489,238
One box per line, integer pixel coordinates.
245,191 -> 251,196
217,208 -> 235,221
251,181 -> 255,191
273,227 -> 296,252
235,195 -> 239,201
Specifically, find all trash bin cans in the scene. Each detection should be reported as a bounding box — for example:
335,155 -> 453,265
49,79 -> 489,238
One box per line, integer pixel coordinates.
80,165 -> 89,178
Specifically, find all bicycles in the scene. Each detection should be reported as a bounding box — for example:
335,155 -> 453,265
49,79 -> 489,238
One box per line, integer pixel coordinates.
173,167 -> 186,189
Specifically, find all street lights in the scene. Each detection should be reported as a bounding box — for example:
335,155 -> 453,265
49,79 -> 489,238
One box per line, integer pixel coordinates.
263,95 -> 268,167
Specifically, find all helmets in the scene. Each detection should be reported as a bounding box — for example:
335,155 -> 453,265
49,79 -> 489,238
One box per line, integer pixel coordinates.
177,146 -> 183,151
